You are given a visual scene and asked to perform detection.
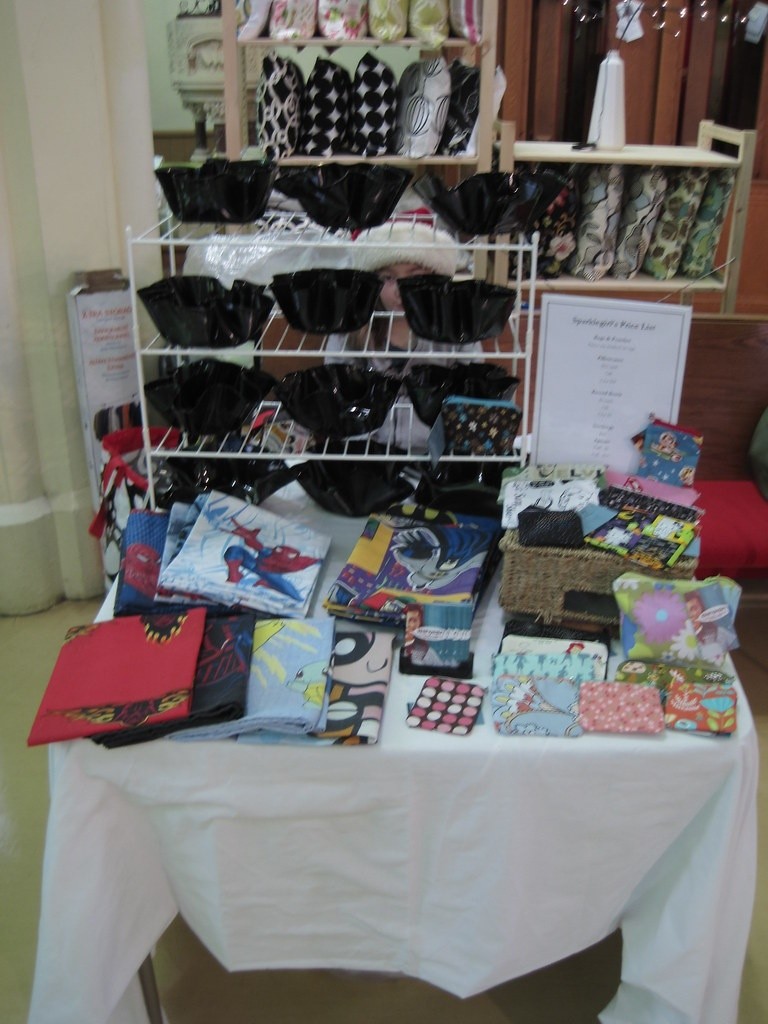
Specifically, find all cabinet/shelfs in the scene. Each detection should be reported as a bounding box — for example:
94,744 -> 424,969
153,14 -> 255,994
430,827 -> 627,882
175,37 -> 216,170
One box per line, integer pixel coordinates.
508,117 -> 756,313
218,0 -> 500,280
124,213 -> 540,509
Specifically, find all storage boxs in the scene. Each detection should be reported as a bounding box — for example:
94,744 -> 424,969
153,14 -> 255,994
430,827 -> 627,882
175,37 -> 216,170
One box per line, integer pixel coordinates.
65,266 -> 141,592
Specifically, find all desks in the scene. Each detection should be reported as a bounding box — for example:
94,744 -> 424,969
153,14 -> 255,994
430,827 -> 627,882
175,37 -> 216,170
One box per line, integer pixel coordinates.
26,480 -> 758,1024
166,19 -> 258,163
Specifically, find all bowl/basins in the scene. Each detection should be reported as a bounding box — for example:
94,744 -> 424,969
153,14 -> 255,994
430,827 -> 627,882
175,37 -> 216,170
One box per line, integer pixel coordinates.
275,363 -> 402,438
156,160 -> 276,223
296,161 -> 413,229
403,361 -> 521,428
165,446 -> 295,507
144,360 -> 277,436
269,268 -> 386,334
415,170 -> 577,234
415,449 -> 530,517
287,459 -> 415,517
396,273 -> 517,344
137,275 -> 274,349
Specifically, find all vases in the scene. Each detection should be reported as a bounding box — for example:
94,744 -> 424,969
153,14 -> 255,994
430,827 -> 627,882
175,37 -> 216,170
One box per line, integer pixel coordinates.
586,49 -> 626,152
